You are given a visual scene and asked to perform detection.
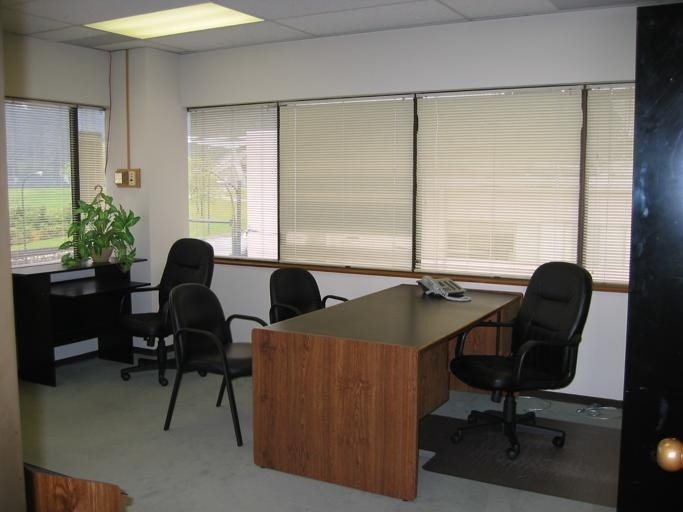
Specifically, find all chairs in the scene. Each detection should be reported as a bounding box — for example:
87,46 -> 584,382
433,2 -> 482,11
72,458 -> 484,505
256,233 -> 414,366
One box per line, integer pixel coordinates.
116,237 -> 216,388
447,261 -> 594,460
166,294 -> 269,448
268,264 -> 350,327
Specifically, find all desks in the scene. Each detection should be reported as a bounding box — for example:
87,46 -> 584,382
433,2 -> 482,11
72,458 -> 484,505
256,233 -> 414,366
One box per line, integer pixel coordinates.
249,284 -> 522,502
9,258 -> 151,387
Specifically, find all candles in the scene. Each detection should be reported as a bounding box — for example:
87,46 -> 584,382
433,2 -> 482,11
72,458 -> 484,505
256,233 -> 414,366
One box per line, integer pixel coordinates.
56,184 -> 141,273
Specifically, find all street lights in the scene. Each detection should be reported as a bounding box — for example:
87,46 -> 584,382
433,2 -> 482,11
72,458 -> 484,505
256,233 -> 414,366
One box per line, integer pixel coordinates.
21,171 -> 43,251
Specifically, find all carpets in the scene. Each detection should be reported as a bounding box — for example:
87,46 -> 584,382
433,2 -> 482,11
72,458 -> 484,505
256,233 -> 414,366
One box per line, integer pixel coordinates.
418,406 -> 622,510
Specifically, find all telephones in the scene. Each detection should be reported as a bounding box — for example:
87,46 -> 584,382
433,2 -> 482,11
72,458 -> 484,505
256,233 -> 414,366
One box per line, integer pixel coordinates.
416,276 -> 466,297
